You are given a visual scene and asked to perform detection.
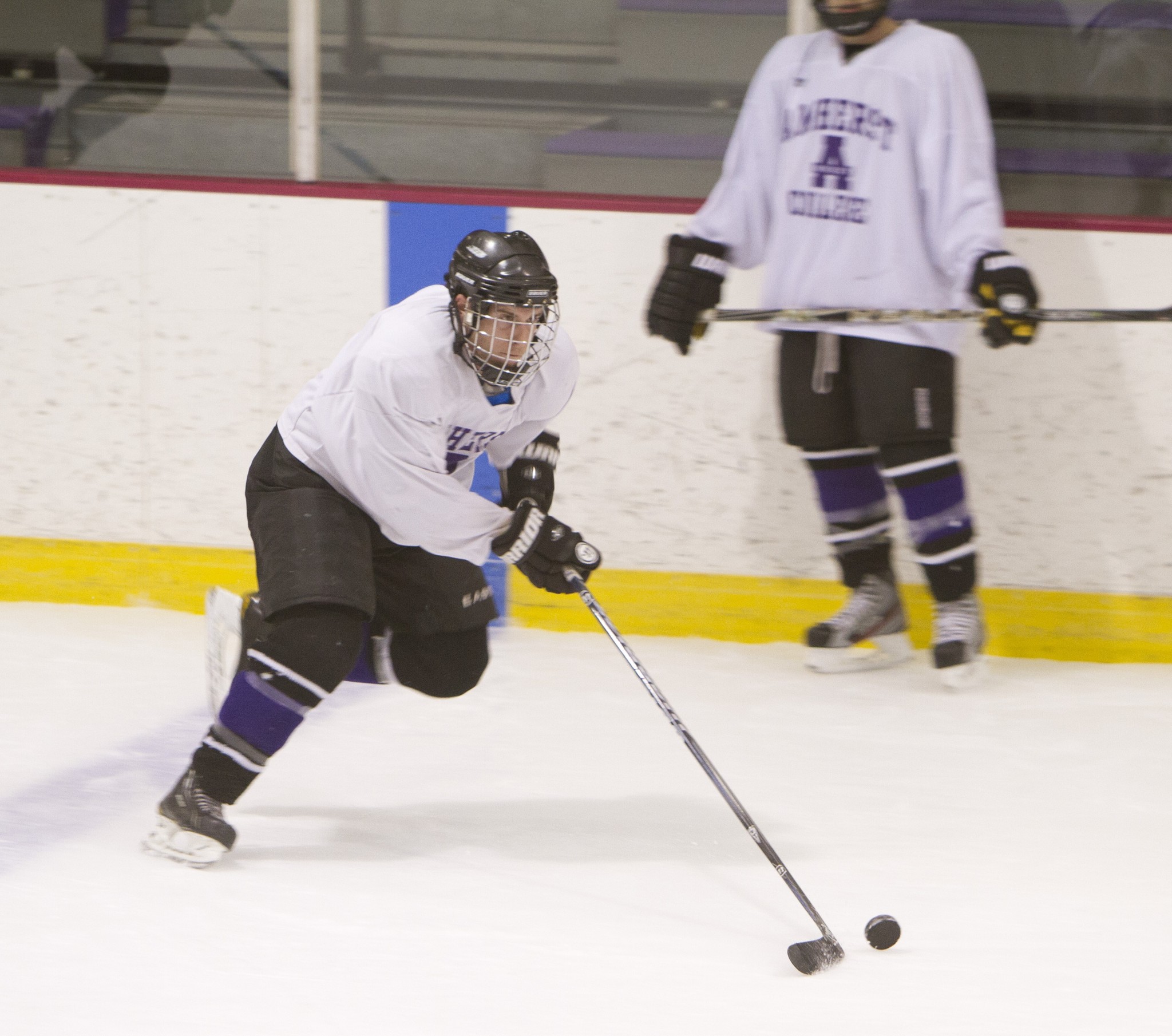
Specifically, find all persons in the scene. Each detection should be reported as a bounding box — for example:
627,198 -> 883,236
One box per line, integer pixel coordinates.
0,0 -> 231,170
647,0 -> 1041,696
143,227 -> 603,866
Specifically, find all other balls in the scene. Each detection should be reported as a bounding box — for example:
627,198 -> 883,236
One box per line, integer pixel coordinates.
864,913 -> 900,951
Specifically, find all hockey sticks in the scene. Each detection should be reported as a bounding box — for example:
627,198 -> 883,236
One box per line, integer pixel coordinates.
195,15 -> 388,185
534,505 -> 847,973
692,302 -> 1168,320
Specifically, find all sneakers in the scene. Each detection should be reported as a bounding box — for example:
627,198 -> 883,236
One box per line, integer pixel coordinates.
801,572 -> 913,678
204,584 -> 246,724
929,588 -> 989,691
145,762 -> 236,866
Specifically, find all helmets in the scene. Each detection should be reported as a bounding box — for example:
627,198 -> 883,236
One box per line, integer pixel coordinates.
444,228 -> 561,390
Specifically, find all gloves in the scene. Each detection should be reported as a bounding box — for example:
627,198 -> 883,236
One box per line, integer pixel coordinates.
967,246 -> 1045,349
506,430 -> 560,518
489,495 -> 600,597
647,233 -> 733,355
